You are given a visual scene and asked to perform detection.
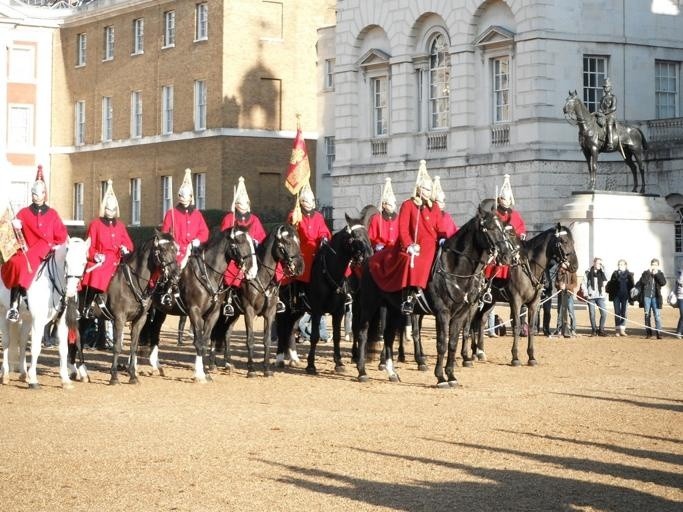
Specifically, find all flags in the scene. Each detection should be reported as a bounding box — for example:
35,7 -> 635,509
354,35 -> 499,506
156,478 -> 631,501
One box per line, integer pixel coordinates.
0,204 -> 30,264
284,125 -> 311,196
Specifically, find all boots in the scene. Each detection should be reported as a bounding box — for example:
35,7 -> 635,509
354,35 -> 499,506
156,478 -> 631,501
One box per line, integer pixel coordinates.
224,284 -> 233,313
657,331 -> 662,339
614,325 -> 627,337
402,286 -> 415,315
83,285 -> 95,319
645,329 -> 651,339
8,285 -> 21,320
160,281 -> 173,310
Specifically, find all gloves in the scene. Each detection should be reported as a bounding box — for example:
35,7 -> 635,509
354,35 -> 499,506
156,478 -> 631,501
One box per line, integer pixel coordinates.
121,244 -> 131,256
94,252 -> 106,263
12,219 -> 22,228
192,238 -> 201,247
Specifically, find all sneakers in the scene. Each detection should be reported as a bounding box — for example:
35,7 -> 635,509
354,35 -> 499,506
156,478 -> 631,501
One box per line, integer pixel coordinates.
344,332 -> 354,342
553,329 -> 579,337
591,331 -> 608,337
297,335 -> 304,343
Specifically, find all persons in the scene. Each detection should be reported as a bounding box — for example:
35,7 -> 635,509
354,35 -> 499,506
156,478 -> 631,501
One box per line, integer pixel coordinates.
512,255 -> 683,339
76,179 -> 134,320
274,187 -> 331,313
1,166 -> 69,321
157,167 -> 209,307
598,76 -> 618,151
353,158 -> 457,319
220,174 -> 283,316
477,175 -> 527,305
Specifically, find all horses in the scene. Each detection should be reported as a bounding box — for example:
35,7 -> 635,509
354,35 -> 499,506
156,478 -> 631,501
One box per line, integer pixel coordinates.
562,90 -> 652,196
1,208 -> 580,391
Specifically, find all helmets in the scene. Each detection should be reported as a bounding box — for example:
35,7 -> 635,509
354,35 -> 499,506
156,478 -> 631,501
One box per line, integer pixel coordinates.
299,185 -> 315,205
31,164 -> 45,197
177,168 -> 195,205
231,176 -> 250,214
413,160 -> 446,203
383,178 -> 396,207
499,174 -> 515,206
98,178 -> 120,218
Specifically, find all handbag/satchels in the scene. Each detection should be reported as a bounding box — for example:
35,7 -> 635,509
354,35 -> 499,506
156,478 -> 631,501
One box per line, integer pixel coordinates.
577,291 -> 587,302
667,291 -> 678,308
